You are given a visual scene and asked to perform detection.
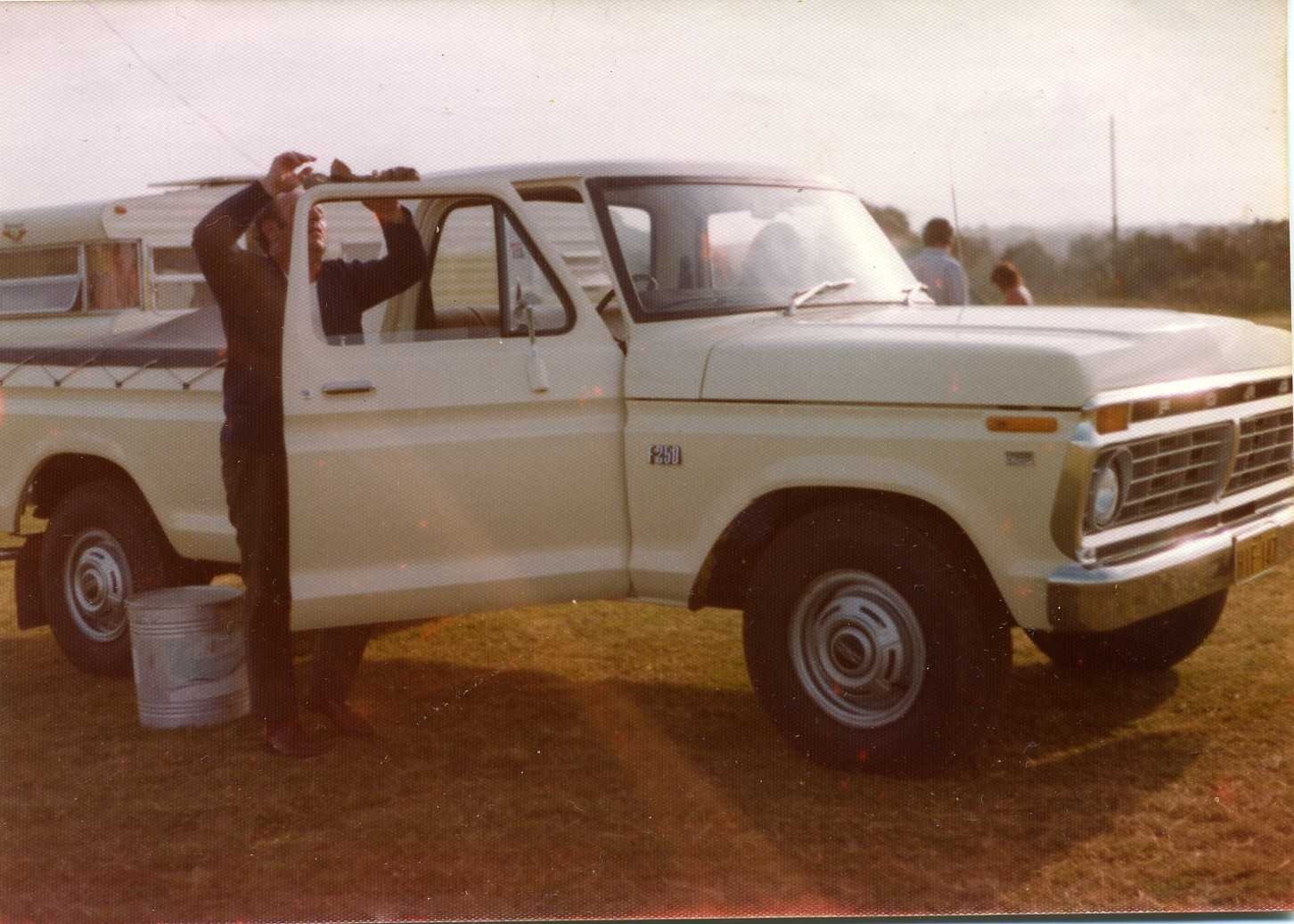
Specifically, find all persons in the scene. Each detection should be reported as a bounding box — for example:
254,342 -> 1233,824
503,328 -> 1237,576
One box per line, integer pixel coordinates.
191,152 -> 428,757
992,260 -> 1034,306
907,218 -> 968,306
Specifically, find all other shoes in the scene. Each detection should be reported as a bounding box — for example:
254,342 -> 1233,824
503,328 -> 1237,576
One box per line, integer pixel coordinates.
304,693 -> 376,736
262,711 -> 309,757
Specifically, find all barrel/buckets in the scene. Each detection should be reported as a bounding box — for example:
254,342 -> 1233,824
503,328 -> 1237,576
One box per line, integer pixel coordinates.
124,586 -> 250,727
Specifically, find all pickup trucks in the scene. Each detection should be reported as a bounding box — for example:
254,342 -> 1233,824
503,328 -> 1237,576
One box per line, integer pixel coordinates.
0,159 -> 1293,777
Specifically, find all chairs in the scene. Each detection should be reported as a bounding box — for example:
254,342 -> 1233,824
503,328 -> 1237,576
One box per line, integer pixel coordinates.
746,224 -> 815,289
